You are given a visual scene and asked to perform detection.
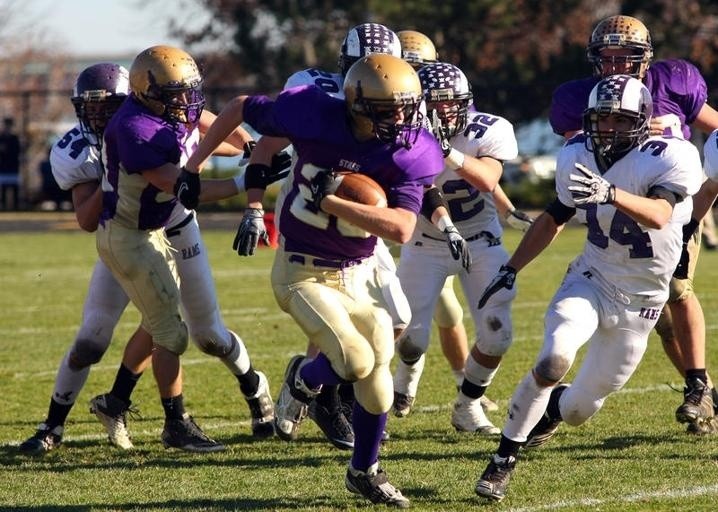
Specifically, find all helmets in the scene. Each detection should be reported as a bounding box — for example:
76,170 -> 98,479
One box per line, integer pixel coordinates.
585,14 -> 653,80
338,23 -> 473,150
71,63 -> 130,145
583,74 -> 653,162
129,46 -> 205,124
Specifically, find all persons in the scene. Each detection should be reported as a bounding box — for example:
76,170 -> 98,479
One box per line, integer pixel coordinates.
2,15 -> 718,507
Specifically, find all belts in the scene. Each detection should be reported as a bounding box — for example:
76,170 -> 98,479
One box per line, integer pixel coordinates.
288,254 -> 367,270
422,232 -> 485,243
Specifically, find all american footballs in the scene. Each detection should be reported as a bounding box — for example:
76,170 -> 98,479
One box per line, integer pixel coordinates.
334,171 -> 388,207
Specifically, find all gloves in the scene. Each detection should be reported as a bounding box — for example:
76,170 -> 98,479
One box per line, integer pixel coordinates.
306,167 -> 334,211
673,244 -> 689,280
478,265 -> 515,309
444,226 -> 473,273
504,210 -> 538,233
568,164 -> 616,206
233,208 -> 270,256
238,140 -> 291,190
173,167 -> 201,209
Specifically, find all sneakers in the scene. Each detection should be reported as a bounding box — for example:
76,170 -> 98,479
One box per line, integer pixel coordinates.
161,413 -> 222,452
90,393 -> 137,451
451,404 -> 502,440
454,383 -> 499,411
676,372 -> 718,435
241,355 -> 390,450
18,421 -> 64,456
392,392 -> 414,417
474,453 -> 516,500
346,459 -> 411,508
522,381 -> 571,447
